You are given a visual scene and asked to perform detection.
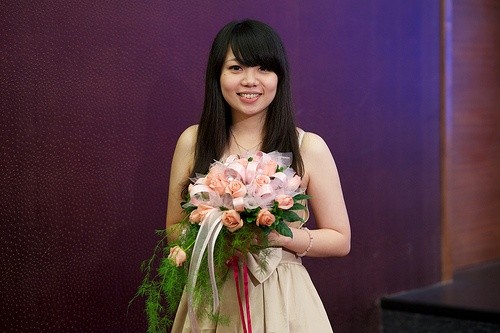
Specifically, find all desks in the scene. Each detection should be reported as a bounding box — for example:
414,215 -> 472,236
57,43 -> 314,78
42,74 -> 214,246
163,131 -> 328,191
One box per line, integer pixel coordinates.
379,258 -> 500,333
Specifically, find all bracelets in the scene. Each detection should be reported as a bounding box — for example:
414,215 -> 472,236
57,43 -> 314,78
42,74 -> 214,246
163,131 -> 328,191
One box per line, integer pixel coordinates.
294,226 -> 314,258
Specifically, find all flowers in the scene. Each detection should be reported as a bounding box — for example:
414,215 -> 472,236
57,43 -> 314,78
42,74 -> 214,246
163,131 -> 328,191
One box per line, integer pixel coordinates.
166,149 -> 307,267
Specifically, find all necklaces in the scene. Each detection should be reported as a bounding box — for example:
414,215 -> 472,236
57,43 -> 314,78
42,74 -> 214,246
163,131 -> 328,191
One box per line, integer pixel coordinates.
229,127 -> 268,154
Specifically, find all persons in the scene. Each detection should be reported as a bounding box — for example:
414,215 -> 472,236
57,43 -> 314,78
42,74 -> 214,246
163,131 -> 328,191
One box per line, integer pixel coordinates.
165,20 -> 351,333
